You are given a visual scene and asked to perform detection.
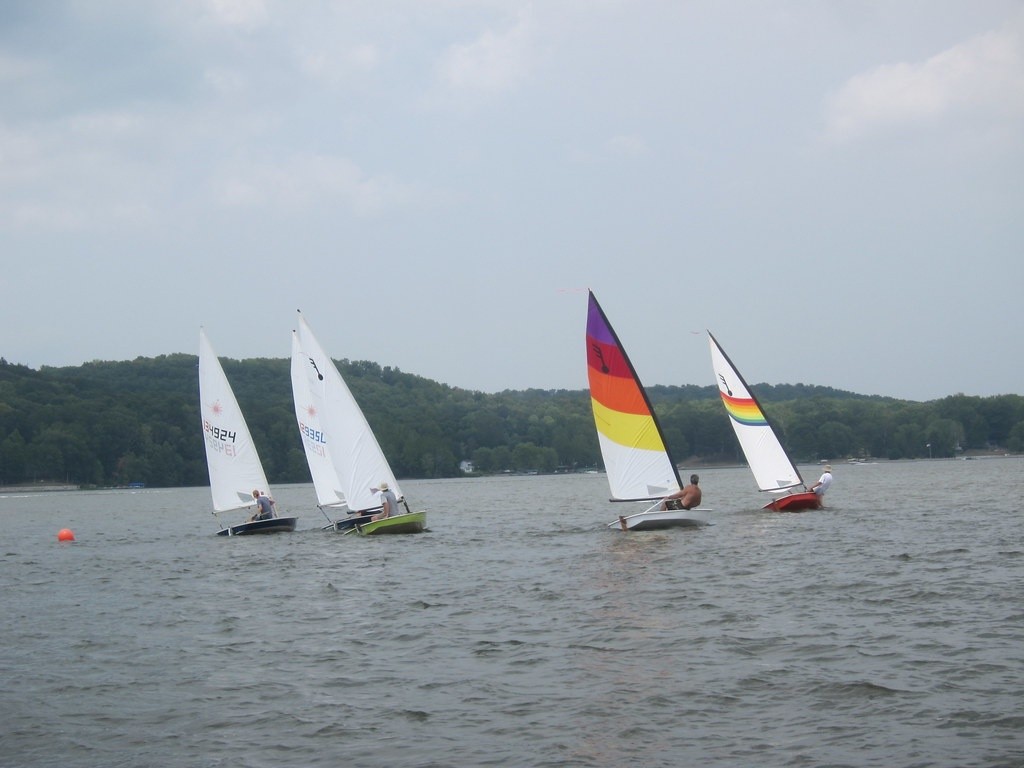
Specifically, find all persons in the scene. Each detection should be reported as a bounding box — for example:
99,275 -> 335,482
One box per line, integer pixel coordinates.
660,474 -> 701,511
370,483 -> 399,522
248,490 -> 276,523
807,465 -> 833,495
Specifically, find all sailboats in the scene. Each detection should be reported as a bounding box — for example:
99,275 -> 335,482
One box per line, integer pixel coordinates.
586,287 -> 714,531
198,326 -> 299,538
290,308 -> 426,537
707,328 -> 817,514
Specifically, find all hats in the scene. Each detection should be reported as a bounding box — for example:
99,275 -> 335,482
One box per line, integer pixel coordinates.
824,465 -> 832,471
381,482 -> 388,490
